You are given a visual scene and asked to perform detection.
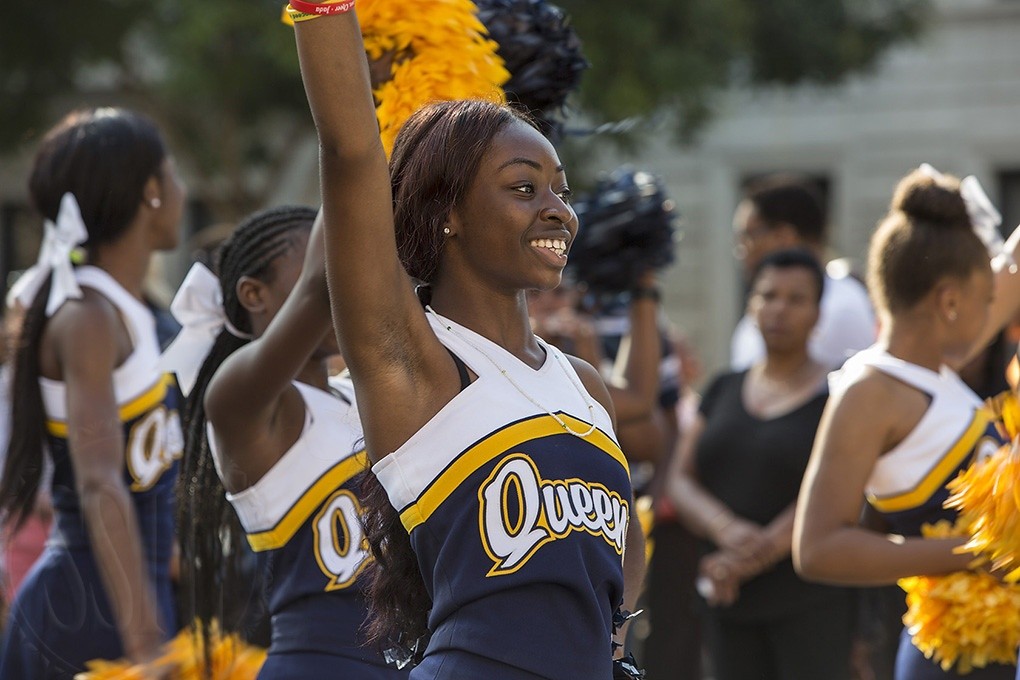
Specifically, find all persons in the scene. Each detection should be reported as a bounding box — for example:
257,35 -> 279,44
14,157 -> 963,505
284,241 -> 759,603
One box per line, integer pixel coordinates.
521,258 -> 706,677
791,165 -> 1020,679
175,199 -> 413,680
665,252 -> 860,678
281,0 -> 650,680
723,169 -> 874,372
519,270 -> 1020,680
0,107 -> 190,680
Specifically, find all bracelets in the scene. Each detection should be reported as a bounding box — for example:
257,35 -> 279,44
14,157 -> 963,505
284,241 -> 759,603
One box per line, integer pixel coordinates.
286,0 -> 355,23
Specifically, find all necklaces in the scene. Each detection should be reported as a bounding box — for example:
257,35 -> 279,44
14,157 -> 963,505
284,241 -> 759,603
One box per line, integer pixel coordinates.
424,303 -> 597,436
